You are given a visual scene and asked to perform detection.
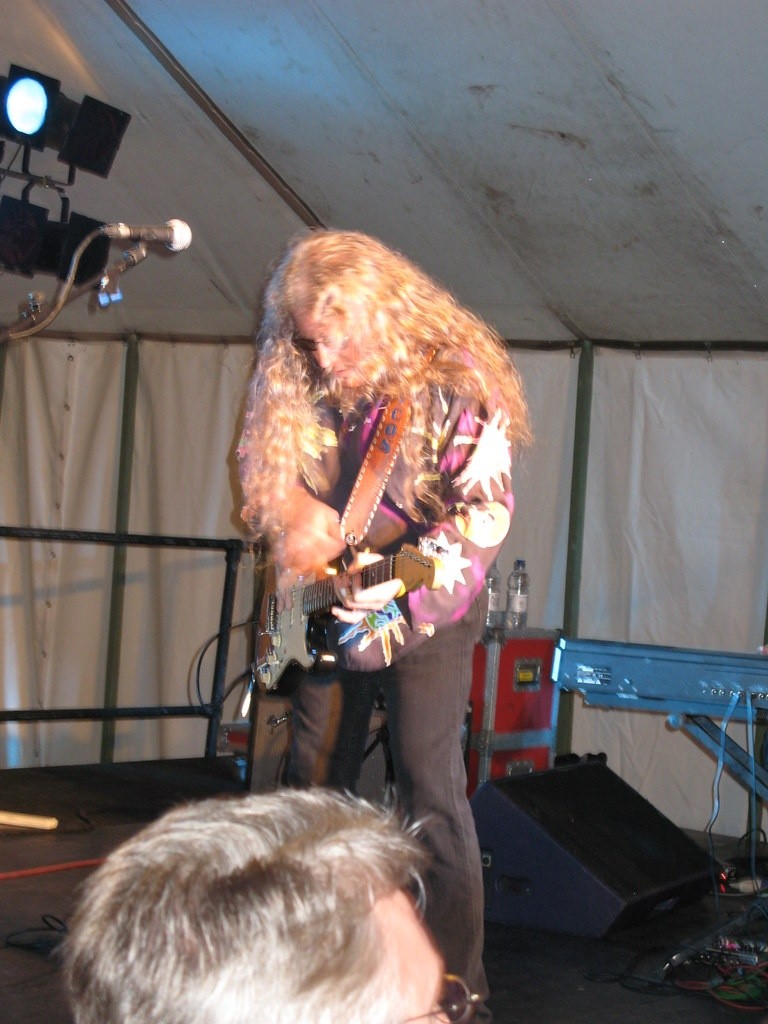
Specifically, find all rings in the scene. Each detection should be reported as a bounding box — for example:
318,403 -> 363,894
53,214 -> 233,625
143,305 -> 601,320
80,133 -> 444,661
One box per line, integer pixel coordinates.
338,588 -> 349,598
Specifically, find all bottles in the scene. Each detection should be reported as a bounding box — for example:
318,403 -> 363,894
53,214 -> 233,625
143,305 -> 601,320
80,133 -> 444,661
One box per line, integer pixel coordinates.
485,560 -> 502,628
505,559 -> 531,630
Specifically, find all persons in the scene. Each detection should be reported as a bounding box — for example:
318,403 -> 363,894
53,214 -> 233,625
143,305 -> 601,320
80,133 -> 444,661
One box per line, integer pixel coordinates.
235,229 -> 534,1024
65,786 -> 453,1024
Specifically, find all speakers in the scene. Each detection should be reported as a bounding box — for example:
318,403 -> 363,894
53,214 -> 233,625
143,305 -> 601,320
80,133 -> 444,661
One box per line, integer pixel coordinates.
470,760 -> 725,942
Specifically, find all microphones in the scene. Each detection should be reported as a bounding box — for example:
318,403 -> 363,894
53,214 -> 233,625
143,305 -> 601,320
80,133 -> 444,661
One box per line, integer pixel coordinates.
100,219 -> 192,253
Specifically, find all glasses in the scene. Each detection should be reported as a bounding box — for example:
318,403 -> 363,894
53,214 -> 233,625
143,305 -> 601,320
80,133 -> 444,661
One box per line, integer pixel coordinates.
404,973 -> 481,1024
292,338 -> 350,351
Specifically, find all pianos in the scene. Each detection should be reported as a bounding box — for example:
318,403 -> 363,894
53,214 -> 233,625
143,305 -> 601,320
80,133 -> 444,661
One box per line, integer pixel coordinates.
553,633 -> 768,724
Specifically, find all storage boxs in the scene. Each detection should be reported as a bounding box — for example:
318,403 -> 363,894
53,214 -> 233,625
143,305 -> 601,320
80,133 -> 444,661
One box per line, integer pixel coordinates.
216,626 -> 565,800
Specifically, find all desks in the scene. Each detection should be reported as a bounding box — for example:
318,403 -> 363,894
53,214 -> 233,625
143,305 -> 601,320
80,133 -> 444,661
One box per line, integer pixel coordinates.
549,638 -> 768,984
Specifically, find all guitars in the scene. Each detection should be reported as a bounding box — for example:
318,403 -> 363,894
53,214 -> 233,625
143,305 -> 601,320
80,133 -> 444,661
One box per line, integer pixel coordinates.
251,534 -> 444,698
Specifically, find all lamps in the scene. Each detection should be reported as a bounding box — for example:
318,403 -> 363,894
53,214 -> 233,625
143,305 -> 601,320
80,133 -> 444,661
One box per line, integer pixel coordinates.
0,65 -> 132,289
